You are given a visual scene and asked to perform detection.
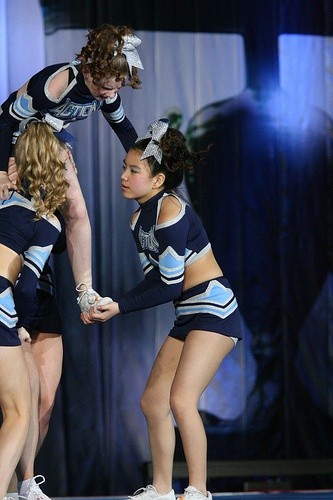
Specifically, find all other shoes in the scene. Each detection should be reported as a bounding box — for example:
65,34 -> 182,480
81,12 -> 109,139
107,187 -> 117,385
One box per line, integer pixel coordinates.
75,281 -> 113,315
2,496 -> 25,500
17,474 -> 53,498
174,483 -> 213,500
128,483 -> 175,499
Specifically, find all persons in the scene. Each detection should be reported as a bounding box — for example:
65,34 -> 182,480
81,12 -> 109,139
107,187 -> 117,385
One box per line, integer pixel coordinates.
0,120 -> 75,500
0,24 -> 144,316
79,118 -> 243,500
